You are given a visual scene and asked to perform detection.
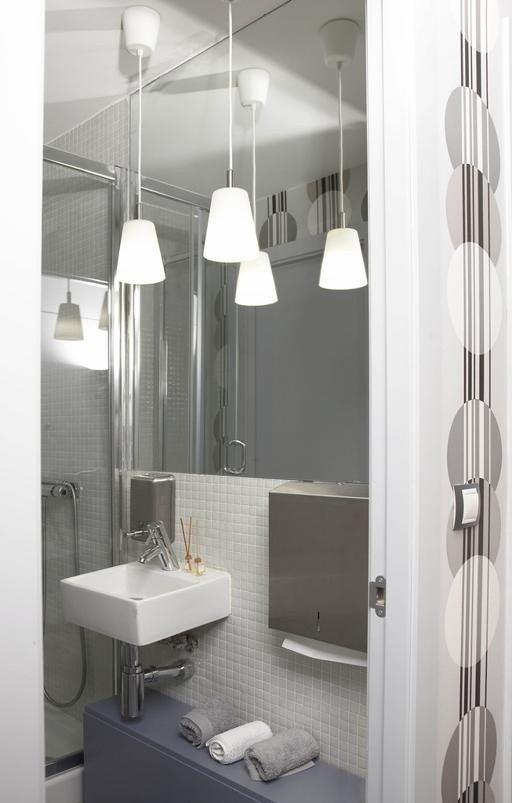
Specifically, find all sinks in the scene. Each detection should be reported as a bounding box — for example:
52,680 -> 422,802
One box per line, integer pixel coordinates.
57,561 -> 234,649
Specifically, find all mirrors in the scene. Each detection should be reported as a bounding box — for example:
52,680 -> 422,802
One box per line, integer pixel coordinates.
131,0 -> 368,484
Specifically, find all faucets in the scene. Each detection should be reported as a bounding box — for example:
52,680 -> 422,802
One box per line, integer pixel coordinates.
123,519 -> 180,572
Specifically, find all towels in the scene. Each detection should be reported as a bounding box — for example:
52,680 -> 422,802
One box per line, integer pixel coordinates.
178,697 -> 234,750
244,728 -> 320,781
205,720 -> 272,764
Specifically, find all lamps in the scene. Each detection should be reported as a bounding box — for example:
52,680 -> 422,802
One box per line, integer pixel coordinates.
112,7 -> 167,286
53,278 -> 86,340
315,17 -> 369,290
234,68 -> 278,307
97,290 -> 112,331
201,1 -> 259,265
